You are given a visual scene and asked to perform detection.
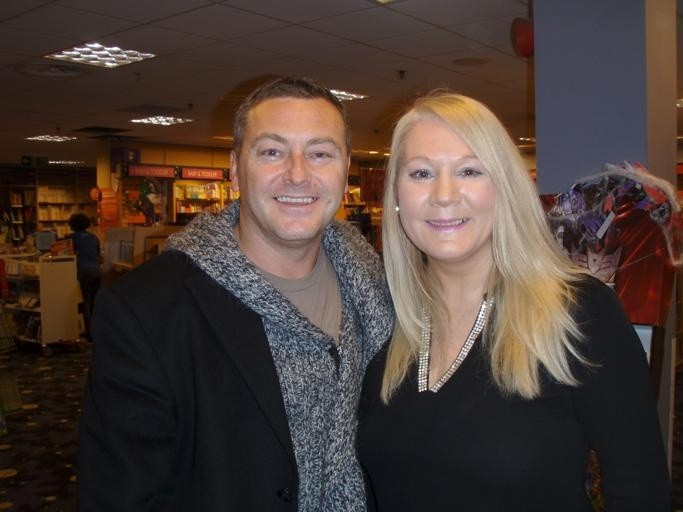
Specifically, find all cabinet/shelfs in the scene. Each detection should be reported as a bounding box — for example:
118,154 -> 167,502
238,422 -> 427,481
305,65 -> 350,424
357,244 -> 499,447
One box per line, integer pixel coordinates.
0,183 -> 223,348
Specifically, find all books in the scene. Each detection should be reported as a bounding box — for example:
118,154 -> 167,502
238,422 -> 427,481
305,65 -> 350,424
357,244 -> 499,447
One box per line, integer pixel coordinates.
175,181 -> 242,213
1,182 -> 91,342
346,182 -> 384,226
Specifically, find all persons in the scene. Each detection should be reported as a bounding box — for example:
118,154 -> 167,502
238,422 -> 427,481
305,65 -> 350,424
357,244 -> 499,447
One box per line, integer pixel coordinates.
65,213 -> 102,340
355,90 -> 669,512
78,76 -> 394,511
